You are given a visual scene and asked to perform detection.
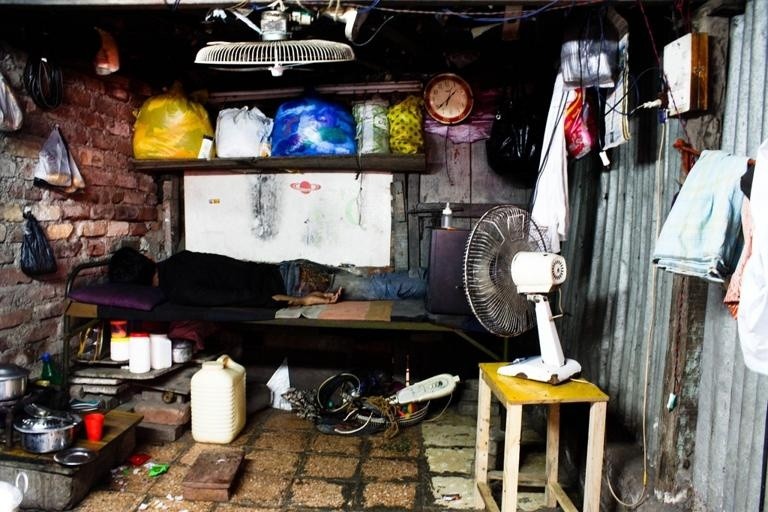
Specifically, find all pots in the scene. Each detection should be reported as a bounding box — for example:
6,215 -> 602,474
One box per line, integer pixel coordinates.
12,410 -> 82,455
0,362 -> 31,402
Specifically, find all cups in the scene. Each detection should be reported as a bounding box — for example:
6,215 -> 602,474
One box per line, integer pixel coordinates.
82,413 -> 106,442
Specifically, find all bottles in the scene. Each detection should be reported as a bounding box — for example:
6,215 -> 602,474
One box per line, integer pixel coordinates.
440,201 -> 455,228
39,354 -> 65,388
111,331 -> 194,375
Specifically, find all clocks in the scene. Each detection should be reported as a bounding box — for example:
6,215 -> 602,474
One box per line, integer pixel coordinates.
425,74 -> 475,125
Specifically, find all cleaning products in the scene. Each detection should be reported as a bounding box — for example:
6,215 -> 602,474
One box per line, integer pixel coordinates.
37,353 -> 59,382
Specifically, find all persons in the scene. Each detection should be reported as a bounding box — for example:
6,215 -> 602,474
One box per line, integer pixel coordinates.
104,247 -> 427,309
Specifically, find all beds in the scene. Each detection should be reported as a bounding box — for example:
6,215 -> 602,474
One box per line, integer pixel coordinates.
63,258 -> 457,404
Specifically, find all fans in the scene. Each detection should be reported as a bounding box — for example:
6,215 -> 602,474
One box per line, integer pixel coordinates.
192,3 -> 355,77
462,203 -> 581,384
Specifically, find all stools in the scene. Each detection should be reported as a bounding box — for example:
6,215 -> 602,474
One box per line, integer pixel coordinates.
475,361 -> 612,511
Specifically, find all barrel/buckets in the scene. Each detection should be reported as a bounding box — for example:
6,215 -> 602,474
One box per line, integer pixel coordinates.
190,355 -> 246,444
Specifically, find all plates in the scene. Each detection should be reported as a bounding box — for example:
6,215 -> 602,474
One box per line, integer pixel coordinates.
53,447 -> 97,467
